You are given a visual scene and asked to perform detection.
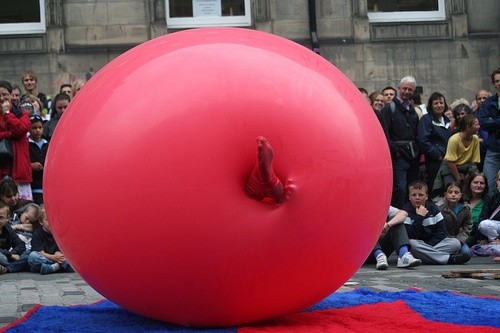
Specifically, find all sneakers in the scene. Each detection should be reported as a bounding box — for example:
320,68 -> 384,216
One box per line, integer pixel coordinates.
397,252 -> 421,268
0,265 -> 7,274
376,253 -> 388,269
449,253 -> 471,265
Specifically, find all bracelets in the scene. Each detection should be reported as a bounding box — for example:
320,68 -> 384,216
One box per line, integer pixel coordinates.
5,111 -> 10,113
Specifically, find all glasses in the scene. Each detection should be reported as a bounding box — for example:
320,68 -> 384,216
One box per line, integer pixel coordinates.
30,116 -> 40,120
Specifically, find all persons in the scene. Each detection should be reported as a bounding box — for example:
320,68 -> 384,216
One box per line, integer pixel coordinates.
358,68 -> 500,271
0,69 -> 77,274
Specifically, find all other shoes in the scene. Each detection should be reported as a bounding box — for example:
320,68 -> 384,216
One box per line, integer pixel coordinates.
41,262 -> 53,275
